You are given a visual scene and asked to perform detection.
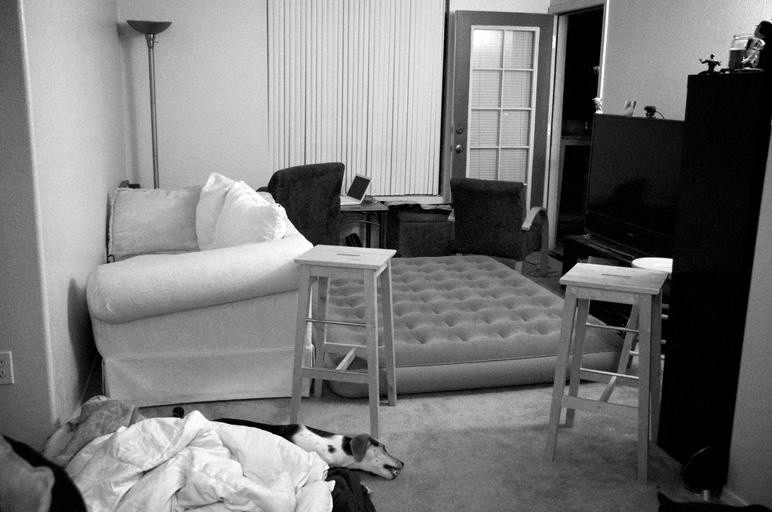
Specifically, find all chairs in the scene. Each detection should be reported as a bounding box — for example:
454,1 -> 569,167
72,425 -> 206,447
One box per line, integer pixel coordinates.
450,177 -> 549,274
256,162 -> 345,244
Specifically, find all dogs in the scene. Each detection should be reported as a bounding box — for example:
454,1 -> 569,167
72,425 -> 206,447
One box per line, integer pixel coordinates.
211,418 -> 404,481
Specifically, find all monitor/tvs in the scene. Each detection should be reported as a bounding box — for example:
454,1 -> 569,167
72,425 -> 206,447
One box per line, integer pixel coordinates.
584,113 -> 686,260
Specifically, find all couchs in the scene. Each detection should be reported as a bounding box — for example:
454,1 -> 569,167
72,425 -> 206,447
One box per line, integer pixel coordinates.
86,187 -> 313,407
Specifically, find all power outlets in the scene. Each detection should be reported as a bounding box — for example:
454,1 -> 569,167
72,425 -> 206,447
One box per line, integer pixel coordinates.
0,350 -> 14,385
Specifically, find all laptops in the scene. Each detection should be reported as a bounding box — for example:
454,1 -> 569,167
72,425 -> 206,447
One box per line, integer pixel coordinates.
340,175 -> 373,205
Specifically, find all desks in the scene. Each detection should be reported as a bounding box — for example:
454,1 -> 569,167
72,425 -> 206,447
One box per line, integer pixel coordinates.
339,200 -> 389,247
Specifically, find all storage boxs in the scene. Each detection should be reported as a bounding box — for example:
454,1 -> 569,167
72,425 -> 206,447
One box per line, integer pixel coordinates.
397,212 -> 455,257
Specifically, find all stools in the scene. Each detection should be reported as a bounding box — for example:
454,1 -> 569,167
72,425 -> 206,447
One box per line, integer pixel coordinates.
290,245 -> 397,441
617,258 -> 673,389
544,263 -> 669,485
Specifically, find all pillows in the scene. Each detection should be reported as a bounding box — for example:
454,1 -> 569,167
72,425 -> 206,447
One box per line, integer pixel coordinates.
213,181 -> 286,249
195,171 -> 235,250
